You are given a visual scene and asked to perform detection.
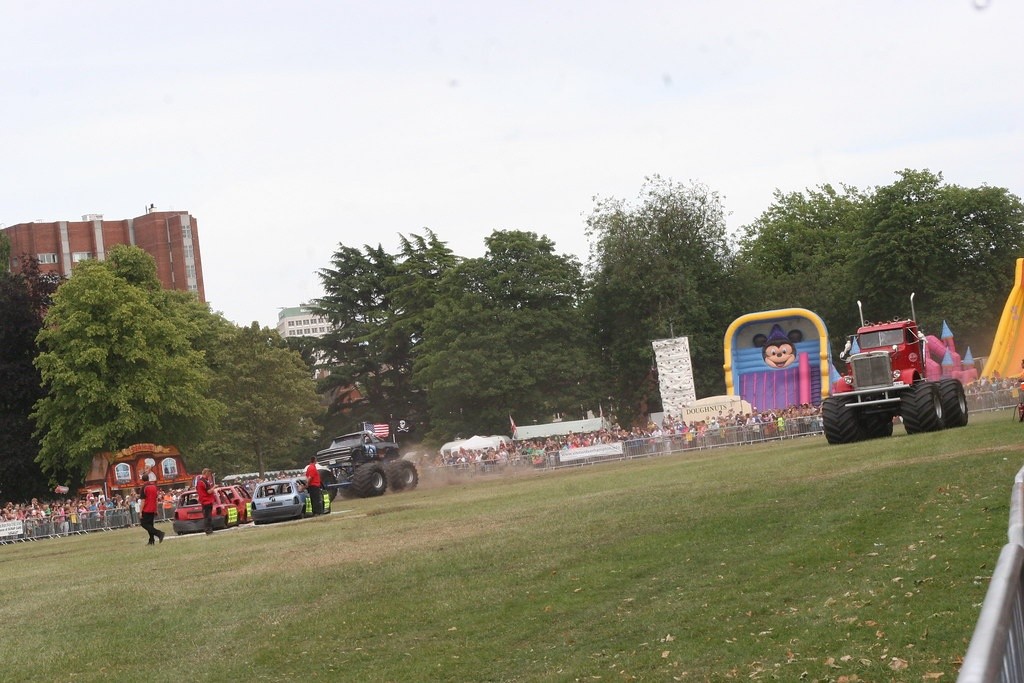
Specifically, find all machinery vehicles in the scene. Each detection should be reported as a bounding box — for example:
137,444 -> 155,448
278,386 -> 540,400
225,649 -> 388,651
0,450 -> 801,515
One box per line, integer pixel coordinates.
314,431 -> 419,498
819,294 -> 969,445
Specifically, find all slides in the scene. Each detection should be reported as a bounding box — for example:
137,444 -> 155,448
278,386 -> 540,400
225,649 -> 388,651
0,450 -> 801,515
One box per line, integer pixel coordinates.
732,353 -> 811,415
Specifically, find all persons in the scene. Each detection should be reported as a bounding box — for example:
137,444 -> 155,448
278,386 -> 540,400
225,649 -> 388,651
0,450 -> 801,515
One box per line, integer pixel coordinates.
154,468 -> 303,523
305,457 -> 321,515
196,468 -> 219,534
0,486 -> 140,539
416,403 -> 824,477
139,474 -> 165,546
964,376 -> 1024,411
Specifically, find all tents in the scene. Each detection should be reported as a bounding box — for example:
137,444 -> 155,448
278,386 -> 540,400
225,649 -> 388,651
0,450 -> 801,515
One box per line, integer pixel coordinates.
438,435 -> 511,461
513,417 -> 613,450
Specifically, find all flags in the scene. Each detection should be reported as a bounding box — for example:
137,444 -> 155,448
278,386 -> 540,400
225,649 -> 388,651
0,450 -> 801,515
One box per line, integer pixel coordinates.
508,414 -> 518,438
364,421 -> 389,440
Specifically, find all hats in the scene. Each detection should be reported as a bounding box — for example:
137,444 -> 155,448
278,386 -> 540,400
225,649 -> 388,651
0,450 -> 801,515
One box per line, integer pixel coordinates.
754,407 -> 757,409
0,511 -> 8,516
90,497 -> 94,499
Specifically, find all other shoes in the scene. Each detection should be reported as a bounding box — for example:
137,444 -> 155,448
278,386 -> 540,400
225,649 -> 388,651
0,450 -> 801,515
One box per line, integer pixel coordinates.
206,529 -> 213,534
159,532 -> 165,543
147,542 -> 154,546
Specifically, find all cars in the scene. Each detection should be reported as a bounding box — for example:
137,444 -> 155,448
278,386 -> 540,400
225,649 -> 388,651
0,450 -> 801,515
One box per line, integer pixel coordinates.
173,485 -> 241,536
213,485 -> 255,524
248,477 -> 308,525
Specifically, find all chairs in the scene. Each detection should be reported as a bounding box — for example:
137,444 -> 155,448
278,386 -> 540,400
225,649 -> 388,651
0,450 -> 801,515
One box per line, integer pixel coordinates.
287,486 -> 291,493
266,488 -> 275,496
229,493 -> 233,498
190,499 -> 197,504
